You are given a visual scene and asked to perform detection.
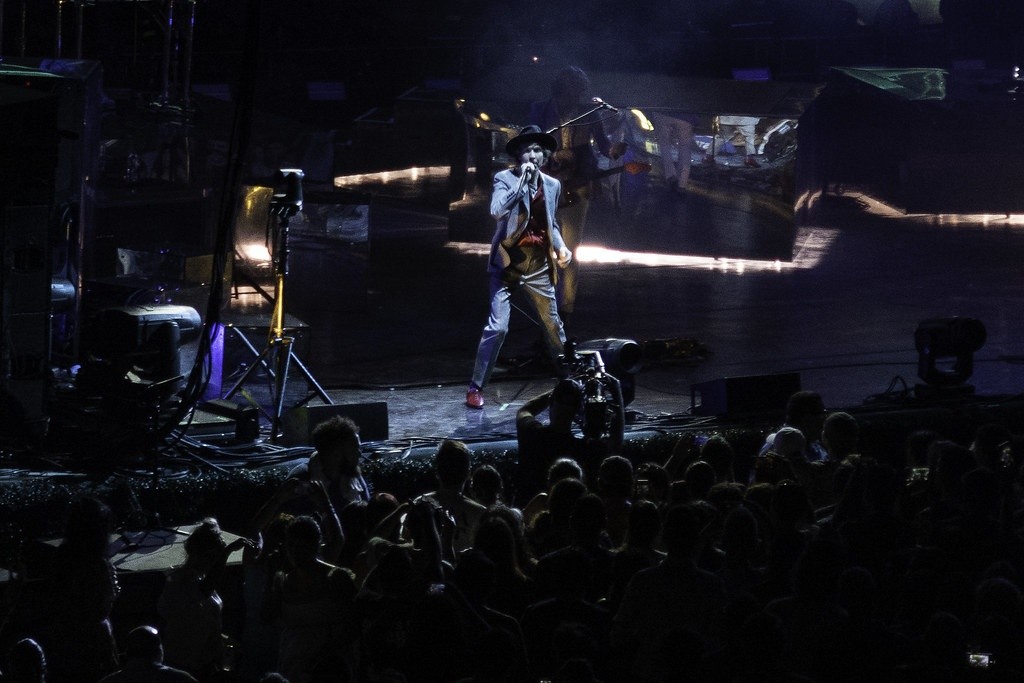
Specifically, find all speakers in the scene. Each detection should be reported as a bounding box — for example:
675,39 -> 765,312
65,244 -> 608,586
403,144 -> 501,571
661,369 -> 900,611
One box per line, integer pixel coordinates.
0,61 -> 86,367
688,371 -> 804,422
277,400 -> 389,447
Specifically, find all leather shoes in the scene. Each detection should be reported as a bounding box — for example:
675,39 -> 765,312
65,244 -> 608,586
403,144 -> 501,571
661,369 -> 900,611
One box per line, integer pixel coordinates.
467,388 -> 484,407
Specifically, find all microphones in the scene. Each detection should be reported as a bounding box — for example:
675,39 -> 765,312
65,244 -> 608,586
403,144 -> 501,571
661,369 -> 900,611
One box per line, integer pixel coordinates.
526,166 -> 531,172
589,95 -> 616,115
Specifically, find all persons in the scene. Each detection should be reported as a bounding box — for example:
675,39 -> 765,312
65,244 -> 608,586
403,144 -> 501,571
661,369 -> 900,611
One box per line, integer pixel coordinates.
467,127 -> 572,409
536,66 -> 625,321
0,377 -> 1024,683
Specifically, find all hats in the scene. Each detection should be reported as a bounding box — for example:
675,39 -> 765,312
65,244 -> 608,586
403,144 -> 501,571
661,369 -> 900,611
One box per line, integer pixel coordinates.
766,426 -> 806,458
505,125 -> 557,158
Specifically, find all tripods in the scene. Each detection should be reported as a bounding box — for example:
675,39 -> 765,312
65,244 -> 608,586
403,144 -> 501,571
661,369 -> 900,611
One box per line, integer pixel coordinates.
222,196 -> 334,420
130,385 -> 191,547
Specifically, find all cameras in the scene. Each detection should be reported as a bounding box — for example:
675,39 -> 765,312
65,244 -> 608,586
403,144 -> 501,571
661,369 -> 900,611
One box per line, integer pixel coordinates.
690,434 -> 710,446
965,652 -> 993,670
909,466 -> 929,481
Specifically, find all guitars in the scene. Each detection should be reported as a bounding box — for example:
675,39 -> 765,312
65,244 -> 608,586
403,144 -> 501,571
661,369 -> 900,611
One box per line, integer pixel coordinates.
557,163 -> 651,209
